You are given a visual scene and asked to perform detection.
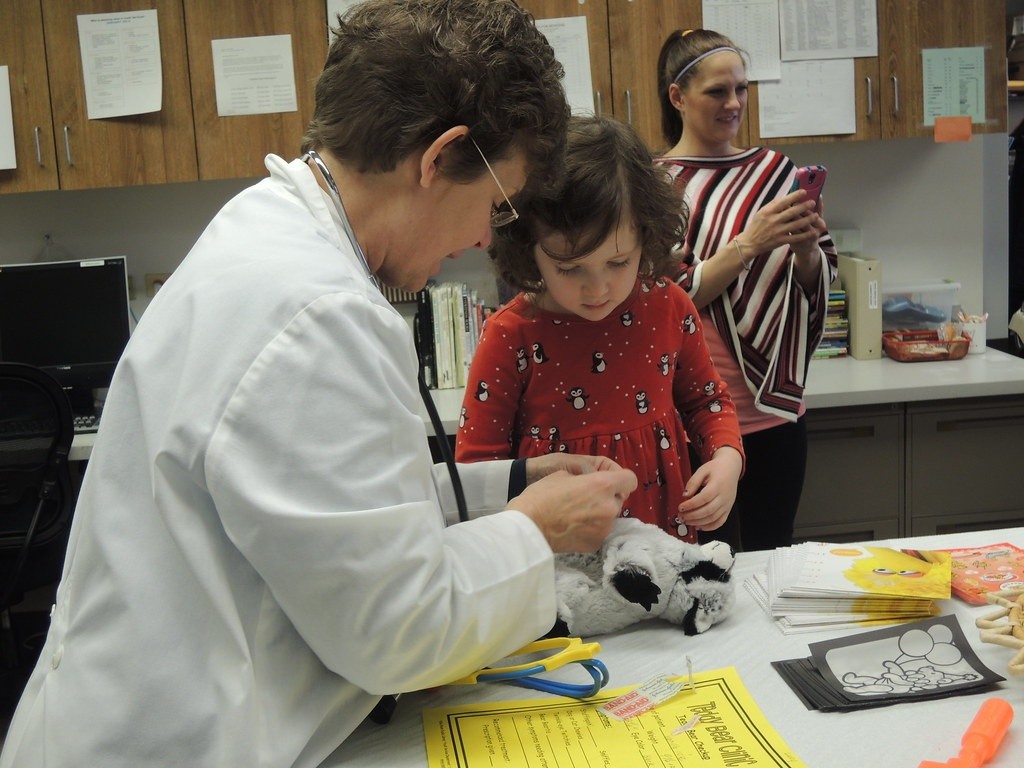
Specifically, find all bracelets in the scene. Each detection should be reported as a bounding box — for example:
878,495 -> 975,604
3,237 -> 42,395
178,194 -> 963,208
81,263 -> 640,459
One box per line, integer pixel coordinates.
734,235 -> 751,271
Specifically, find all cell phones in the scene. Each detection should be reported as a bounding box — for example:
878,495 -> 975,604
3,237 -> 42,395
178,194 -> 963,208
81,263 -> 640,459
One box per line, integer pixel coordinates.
787,165 -> 828,219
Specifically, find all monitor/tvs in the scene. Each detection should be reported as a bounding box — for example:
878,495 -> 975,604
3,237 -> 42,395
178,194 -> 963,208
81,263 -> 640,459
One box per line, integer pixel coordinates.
0,257 -> 132,410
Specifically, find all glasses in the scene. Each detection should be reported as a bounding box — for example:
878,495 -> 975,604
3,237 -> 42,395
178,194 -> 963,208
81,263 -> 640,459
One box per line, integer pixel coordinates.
468,134 -> 519,227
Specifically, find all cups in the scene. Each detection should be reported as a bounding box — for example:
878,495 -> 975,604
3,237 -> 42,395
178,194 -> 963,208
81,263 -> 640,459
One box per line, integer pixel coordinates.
958,321 -> 986,354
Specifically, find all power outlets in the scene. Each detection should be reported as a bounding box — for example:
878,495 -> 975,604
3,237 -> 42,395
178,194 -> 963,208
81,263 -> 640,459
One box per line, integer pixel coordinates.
146,272 -> 167,297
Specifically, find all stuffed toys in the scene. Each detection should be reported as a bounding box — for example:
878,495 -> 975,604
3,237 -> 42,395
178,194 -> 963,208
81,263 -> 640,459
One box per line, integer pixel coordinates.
537,519 -> 737,641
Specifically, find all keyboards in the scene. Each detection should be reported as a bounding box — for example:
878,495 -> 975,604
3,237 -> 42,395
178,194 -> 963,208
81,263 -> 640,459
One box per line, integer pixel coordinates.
73,415 -> 103,434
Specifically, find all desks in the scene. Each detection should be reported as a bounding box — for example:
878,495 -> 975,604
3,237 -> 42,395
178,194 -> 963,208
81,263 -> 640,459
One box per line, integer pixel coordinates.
325,525 -> 1024,768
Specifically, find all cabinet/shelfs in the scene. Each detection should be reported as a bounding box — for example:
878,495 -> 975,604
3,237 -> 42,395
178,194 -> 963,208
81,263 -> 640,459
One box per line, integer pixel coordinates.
0,343 -> 1023,546
0,0 -> 1008,196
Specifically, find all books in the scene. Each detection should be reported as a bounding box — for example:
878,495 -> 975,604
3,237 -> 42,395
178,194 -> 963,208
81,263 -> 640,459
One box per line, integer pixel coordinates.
417,280 -> 497,390
743,542 -> 1024,635
811,289 -> 849,359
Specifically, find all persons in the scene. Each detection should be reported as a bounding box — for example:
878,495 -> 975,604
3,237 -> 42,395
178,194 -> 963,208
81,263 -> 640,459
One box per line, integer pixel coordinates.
455,116 -> 747,545
646,27 -> 838,550
0,0 -> 639,768
